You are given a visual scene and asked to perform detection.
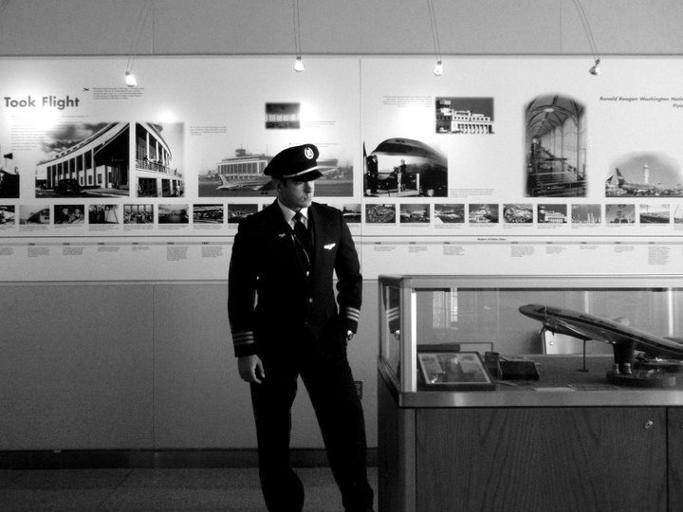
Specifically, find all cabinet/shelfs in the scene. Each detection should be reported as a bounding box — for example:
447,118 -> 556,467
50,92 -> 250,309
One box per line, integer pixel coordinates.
378,275 -> 683,512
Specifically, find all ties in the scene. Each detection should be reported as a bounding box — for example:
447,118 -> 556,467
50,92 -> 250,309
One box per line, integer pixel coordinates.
291,210 -> 313,265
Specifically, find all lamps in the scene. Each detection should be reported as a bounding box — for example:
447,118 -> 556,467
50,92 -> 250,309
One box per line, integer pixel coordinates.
124,1 -> 154,88
426,1 -> 443,76
573,0 -> 602,75
290,0 -> 305,72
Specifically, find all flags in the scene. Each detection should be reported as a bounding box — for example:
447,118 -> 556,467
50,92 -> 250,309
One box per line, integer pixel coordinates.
3,152 -> 12,160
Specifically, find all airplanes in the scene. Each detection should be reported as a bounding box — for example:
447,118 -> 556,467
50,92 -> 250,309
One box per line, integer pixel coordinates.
615,168 -> 673,196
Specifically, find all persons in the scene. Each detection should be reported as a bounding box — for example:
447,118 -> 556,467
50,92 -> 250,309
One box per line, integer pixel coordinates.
88,205 -> 152,224
225,140 -> 377,512
56,207 -> 72,223
430,356 -> 475,383
393,160 -> 406,193
367,155 -> 380,194
178,209 -> 188,223
68,207 -> 83,223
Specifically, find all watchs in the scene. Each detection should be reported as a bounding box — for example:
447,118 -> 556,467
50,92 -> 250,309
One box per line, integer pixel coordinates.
345,328 -> 354,340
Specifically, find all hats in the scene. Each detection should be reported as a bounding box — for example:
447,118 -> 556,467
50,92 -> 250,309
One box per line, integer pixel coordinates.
261,144 -> 322,184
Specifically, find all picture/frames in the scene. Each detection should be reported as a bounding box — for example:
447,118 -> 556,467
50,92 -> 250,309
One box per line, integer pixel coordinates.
417,350 -> 496,392
